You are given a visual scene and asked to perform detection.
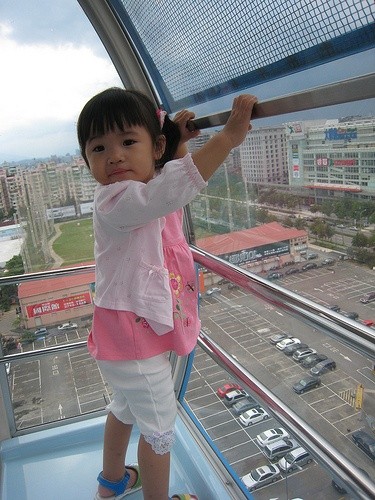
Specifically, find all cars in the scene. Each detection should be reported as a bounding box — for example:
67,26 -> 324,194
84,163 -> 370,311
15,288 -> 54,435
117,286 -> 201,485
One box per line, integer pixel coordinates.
231,399 -> 259,414
215,382 -> 243,399
287,214 -> 362,230
240,409 -> 269,425
263,248 -> 343,280
55,321 -> 79,330
353,428 -> 375,457
311,360 -> 337,376
293,375 -> 321,395
204,276 -> 243,297
258,428 -> 289,447
32,328 -> 50,336
300,354 -> 328,367
239,465 -> 282,491
271,333 -> 316,363
329,289 -> 375,329
220,388 -> 248,399
48,208 -> 65,218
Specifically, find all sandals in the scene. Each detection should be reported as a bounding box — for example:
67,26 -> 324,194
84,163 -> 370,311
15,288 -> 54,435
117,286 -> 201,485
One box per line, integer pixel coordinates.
95,462 -> 142,500
168,493 -> 198,500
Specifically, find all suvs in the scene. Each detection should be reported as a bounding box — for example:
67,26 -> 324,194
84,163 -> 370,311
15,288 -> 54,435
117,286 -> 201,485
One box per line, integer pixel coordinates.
277,448 -> 312,474
264,439 -> 301,461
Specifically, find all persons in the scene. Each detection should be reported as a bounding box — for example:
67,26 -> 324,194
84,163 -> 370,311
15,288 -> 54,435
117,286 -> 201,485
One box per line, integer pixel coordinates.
77,87 -> 256,500
